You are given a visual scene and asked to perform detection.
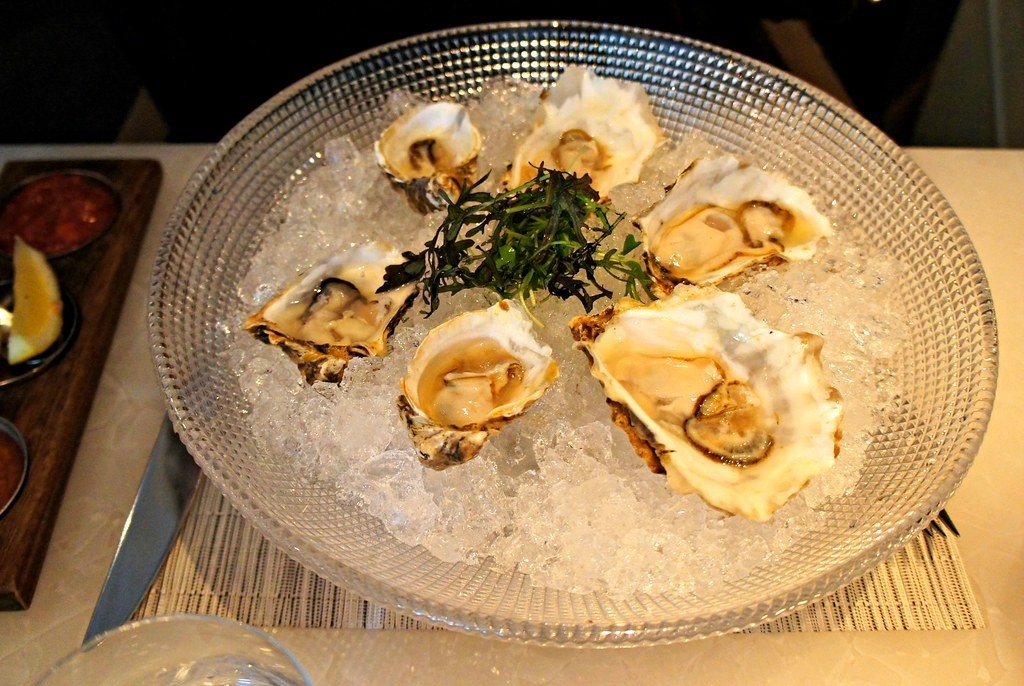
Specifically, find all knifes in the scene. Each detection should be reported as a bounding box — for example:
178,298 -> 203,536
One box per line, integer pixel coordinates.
77,408 -> 200,648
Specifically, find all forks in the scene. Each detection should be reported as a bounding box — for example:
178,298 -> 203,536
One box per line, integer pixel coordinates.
921,506 -> 960,539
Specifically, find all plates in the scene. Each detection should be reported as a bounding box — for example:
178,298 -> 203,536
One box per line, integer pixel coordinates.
0,277 -> 78,388
0,417 -> 27,517
0,168 -> 122,261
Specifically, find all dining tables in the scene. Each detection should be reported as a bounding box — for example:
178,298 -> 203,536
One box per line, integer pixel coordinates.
0,145 -> 1024,685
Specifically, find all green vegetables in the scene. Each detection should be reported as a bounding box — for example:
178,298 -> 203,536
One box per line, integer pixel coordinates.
376,144 -> 661,326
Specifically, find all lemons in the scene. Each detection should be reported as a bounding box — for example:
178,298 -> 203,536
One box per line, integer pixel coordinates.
7,236 -> 63,366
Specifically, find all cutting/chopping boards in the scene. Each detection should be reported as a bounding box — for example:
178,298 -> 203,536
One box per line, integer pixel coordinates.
0,155 -> 163,611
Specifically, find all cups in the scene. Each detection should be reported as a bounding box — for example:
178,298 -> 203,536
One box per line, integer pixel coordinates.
35,611 -> 312,685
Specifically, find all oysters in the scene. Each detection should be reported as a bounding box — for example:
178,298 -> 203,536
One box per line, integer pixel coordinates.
242,65 -> 848,524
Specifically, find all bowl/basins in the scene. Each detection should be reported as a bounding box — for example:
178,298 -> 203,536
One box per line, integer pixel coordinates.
145,20 -> 1001,648
